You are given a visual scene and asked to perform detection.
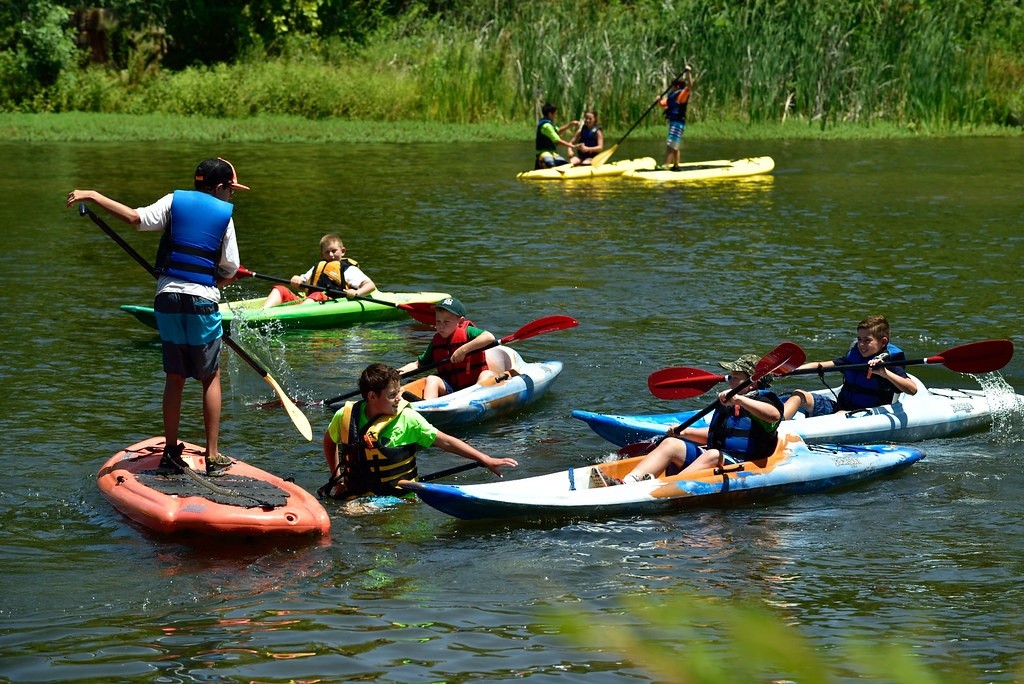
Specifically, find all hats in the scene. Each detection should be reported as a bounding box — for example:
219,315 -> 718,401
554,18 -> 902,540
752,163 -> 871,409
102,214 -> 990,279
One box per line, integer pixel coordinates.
718,354 -> 774,387
435,297 -> 466,316
194,158 -> 250,190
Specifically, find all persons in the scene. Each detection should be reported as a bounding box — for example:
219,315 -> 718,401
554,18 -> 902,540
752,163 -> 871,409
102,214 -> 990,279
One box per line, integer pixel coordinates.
780,314 -> 918,421
567,111 -> 605,165
589,353 -> 785,487
534,102 -> 582,168
655,65 -> 694,171
262,232 -> 376,310
66,157 -> 252,474
394,296 -> 495,403
324,362 -> 518,500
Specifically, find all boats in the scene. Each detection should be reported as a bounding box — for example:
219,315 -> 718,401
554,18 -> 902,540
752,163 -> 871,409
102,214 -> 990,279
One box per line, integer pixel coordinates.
364,343 -> 564,428
572,385 -> 1024,448
118,288 -> 452,334
397,444 -> 926,518
516,155 -> 656,179
623,156 -> 775,182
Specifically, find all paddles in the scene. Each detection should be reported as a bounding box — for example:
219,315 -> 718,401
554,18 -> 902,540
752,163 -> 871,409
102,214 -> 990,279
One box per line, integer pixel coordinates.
590,63 -> 693,165
78,202 -> 314,442
616,342 -> 807,459
419,462 -> 481,482
234,264 -> 436,327
257,314 -> 581,410
648,339 -> 1014,401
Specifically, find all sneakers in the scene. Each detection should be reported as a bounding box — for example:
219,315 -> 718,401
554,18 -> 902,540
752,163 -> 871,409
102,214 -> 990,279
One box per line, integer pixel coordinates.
158,443 -> 190,474
206,453 -> 233,476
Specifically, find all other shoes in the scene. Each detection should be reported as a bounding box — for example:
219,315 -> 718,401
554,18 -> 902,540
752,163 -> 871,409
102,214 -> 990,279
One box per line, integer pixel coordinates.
656,165 -> 666,169
670,166 -> 682,172
641,473 -> 656,480
592,466 -> 626,488
534,153 -> 540,170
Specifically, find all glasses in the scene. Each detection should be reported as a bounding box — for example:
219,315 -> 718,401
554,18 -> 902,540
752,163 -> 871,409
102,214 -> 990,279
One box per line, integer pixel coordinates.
220,183 -> 235,195
